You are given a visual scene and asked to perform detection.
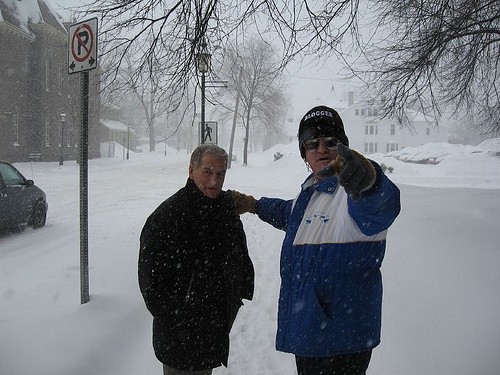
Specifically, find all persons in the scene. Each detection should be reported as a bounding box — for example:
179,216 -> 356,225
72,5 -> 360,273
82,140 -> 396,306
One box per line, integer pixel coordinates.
137,144 -> 255,375
226,105 -> 401,375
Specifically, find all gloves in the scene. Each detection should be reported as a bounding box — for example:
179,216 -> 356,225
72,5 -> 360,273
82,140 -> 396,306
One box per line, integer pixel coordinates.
316,142 -> 376,196
231,190 -> 255,216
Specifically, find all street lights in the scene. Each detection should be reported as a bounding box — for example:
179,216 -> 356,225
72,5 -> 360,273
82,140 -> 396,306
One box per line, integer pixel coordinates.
126,122 -> 132,159
195,41 -> 210,144
59,111 -> 67,165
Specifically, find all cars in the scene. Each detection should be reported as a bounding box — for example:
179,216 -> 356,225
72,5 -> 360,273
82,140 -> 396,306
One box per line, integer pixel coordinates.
0,161 -> 48,233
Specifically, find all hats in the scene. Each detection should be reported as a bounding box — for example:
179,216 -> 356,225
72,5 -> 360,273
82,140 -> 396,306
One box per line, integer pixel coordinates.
298,106 -> 349,157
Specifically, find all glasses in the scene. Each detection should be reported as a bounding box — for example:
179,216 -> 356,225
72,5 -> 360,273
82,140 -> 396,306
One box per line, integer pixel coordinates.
302,135 -> 340,152
